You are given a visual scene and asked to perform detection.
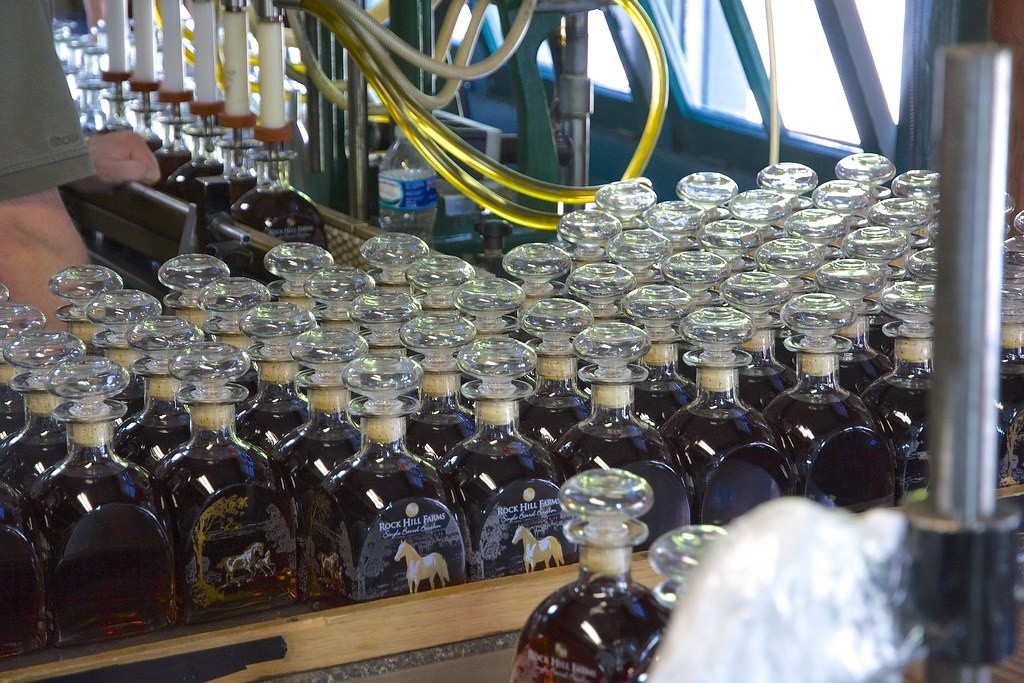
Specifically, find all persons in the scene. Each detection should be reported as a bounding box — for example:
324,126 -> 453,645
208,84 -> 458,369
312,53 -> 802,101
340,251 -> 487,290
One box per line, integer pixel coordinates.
0,2 -> 161,333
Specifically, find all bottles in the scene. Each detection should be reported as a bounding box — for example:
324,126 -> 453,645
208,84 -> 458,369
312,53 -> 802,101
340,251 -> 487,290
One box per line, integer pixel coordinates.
4,3 -> 1023,681
